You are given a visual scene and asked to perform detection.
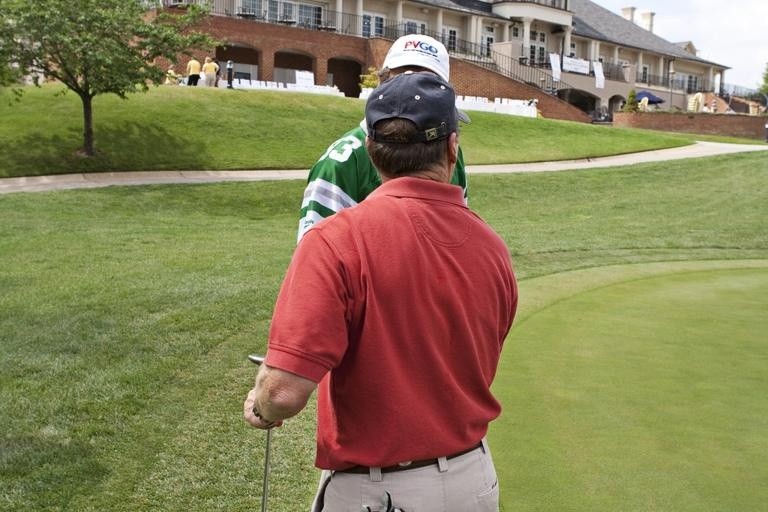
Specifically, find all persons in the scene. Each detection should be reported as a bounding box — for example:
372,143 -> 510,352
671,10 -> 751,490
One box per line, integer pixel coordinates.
295,34 -> 472,242
165,54 -> 221,88
702,102 -> 710,112
242,71 -> 518,512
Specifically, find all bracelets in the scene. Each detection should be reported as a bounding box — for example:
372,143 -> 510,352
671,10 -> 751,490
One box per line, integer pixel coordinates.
252,403 -> 275,425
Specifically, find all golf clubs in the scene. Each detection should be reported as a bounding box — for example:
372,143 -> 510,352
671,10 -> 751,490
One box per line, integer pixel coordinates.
247,354 -> 271,512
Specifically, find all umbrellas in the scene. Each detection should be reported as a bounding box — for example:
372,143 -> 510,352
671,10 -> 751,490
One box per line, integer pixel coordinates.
633,91 -> 665,104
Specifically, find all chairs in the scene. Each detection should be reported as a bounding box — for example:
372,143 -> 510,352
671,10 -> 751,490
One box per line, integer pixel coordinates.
456,94 -> 538,106
257,10 -> 268,21
232,78 -> 284,89
686,92 -> 702,114
225,8 -> 232,16
637,96 -> 649,110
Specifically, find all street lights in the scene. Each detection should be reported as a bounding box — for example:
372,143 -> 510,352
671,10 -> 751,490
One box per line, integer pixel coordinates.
667,70 -> 677,112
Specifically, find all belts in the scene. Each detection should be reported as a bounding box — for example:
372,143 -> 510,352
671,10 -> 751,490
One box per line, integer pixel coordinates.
341,442 -> 482,474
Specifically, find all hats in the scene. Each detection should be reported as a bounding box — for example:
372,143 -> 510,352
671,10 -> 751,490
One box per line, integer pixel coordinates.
364,70 -> 472,144
381,33 -> 451,82
